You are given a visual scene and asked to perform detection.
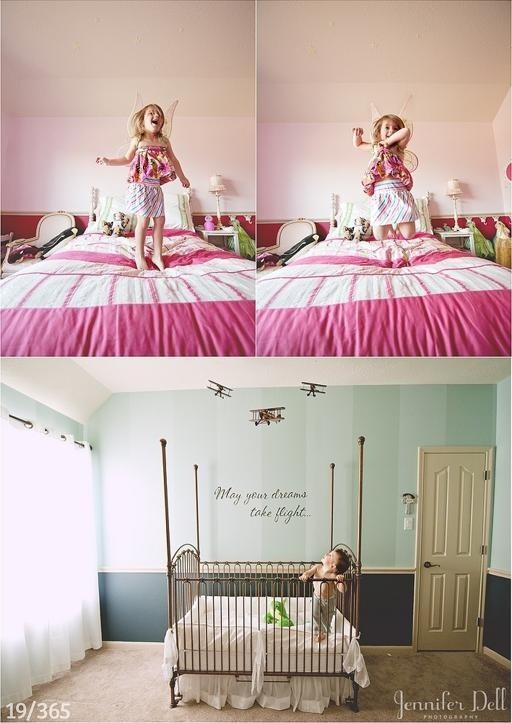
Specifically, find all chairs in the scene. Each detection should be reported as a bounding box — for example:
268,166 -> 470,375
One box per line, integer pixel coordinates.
0,208 -> 75,280
256,217 -> 318,275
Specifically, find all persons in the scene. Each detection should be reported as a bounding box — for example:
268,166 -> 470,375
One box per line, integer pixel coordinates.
351,112 -> 420,241
102,219 -> 114,236
297,546 -> 351,642
95,100 -> 192,272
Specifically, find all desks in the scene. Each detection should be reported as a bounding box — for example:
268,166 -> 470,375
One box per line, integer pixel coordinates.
435,228 -> 478,256
196,227 -> 239,256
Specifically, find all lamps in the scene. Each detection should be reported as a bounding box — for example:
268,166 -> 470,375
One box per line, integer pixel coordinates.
207,174 -> 227,229
445,178 -> 465,230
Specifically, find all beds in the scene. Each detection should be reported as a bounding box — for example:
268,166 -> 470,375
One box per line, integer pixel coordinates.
256,192 -> 512,356
0,185 -> 256,355
160,436 -> 368,712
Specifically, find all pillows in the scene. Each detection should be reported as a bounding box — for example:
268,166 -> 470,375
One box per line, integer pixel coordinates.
81,185 -> 197,235
325,192 -> 434,240
